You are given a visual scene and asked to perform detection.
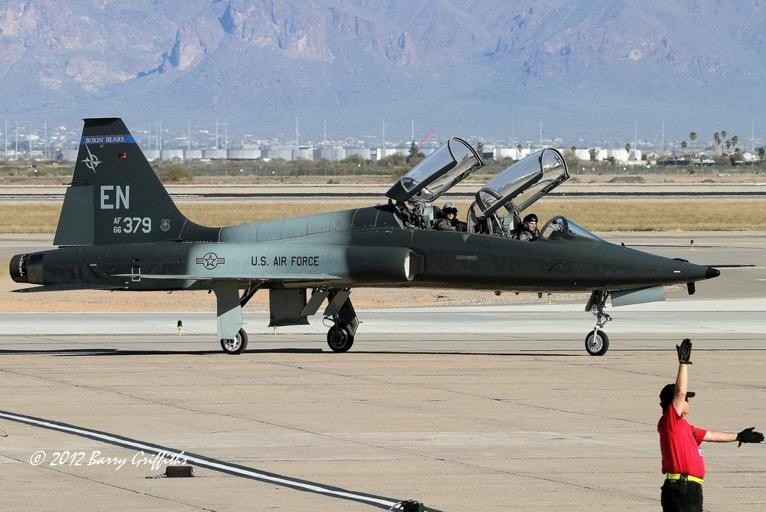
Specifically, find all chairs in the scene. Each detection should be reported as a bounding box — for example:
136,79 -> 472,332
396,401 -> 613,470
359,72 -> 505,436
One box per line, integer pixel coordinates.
497,216 -> 521,240
422,200 -> 441,229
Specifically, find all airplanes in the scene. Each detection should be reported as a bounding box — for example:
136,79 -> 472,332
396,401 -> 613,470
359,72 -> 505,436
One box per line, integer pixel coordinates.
9,118 -> 757,356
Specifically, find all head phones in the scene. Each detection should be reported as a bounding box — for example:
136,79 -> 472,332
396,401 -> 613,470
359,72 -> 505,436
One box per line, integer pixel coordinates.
524,221 -> 529,228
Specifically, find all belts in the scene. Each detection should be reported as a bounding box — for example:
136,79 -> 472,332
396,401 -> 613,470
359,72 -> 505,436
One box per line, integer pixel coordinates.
666,473 -> 704,485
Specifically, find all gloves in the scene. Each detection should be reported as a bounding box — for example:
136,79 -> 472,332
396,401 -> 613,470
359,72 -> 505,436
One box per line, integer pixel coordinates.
676,338 -> 692,364
736,427 -> 765,443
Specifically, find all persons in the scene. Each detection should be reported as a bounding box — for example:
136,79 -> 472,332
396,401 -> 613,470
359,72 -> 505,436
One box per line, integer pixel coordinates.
657,338 -> 765,512
436,203 -> 459,231
519,213 -> 538,241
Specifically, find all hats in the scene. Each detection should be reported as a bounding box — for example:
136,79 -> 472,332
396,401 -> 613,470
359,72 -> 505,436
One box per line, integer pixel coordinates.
659,384 -> 696,409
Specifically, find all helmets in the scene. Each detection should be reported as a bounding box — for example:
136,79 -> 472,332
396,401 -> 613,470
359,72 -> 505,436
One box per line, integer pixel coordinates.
442,203 -> 458,212
523,214 -> 538,224
